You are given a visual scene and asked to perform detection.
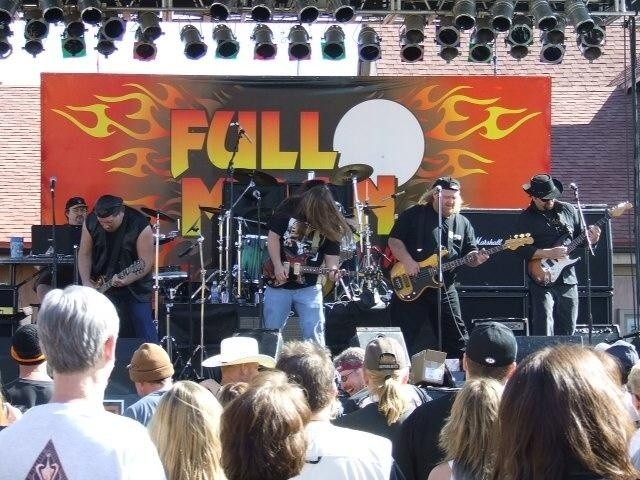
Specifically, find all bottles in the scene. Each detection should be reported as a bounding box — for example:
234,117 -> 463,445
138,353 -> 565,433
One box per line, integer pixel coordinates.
210,280 -> 221,304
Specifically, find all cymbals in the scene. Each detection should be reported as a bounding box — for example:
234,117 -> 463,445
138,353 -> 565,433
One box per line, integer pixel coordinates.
200,205 -> 226,215
328,165 -> 372,186
349,204 -> 386,210
234,167 -> 277,187
141,207 -> 174,224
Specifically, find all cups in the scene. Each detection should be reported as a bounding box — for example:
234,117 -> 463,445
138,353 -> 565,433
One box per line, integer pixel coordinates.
221,292 -> 230,303
10,237 -> 24,259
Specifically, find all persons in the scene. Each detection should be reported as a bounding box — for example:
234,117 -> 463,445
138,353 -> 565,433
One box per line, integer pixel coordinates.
264,186 -> 350,349
33,196 -> 89,289
78,195 -> 159,341
388,177 -> 489,370
0,285 -> 640,480
512,173 -> 602,337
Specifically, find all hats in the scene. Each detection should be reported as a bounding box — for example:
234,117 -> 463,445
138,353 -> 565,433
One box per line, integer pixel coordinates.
65,196 -> 89,212
595,340 -> 639,375
521,173 -> 564,201
128,341 -> 179,384
465,320 -> 517,366
201,335 -> 277,371
9,325 -> 49,365
93,195 -> 123,218
431,176 -> 462,192
363,335 -> 411,372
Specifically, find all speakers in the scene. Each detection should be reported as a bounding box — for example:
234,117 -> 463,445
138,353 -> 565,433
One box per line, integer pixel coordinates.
234,330 -> 283,360
457,207 -> 526,291
569,209 -> 614,292
514,335 -> 582,365
341,326 -> 413,372
570,292 -> 613,325
572,325 -> 619,344
0,285 -> 16,336
455,290 -> 529,337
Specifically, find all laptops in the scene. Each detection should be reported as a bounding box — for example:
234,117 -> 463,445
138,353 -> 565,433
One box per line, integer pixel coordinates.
32,224 -> 82,255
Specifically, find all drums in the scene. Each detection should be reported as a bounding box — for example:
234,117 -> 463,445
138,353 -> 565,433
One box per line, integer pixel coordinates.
154,272 -> 189,304
240,234 -> 269,283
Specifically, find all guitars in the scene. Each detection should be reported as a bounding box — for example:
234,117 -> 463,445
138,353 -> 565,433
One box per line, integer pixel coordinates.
528,202 -> 633,285
262,245 -> 372,287
390,232 -> 534,301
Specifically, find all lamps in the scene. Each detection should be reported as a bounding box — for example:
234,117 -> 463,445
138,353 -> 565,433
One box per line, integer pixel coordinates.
0,0 -> 613,64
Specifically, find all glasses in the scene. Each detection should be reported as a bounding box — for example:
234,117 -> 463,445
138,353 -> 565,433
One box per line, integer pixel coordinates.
340,369 -> 359,382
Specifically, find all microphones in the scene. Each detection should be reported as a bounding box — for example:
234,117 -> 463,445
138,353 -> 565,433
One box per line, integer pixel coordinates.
48,176 -> 56,192
571,183 -> 578,191
242,131 -> 252,143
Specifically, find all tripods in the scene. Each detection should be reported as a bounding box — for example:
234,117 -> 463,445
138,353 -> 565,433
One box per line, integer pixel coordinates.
144,231 -> 248,376
